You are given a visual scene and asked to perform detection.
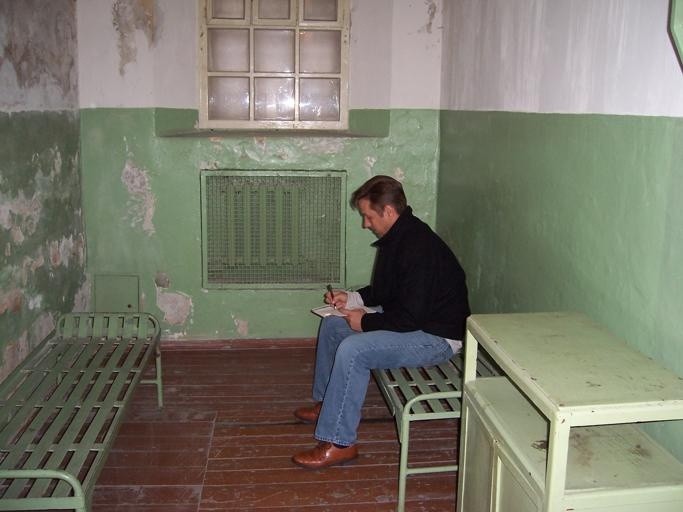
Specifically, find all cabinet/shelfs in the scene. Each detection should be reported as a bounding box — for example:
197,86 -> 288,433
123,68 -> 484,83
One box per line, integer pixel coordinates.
456,312 -> 683,512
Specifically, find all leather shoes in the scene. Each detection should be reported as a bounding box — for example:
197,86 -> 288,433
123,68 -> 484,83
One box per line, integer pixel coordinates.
291,442 -> 357,469
293,403 -> 323,425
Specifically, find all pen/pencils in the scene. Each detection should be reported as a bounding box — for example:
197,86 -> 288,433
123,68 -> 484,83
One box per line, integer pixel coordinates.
327,284 -> 336,310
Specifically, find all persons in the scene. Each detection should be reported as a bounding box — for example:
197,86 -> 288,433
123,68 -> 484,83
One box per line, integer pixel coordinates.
290,174 -> 471,469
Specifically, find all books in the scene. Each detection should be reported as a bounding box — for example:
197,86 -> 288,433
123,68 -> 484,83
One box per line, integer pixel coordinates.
310,304 -> 377,318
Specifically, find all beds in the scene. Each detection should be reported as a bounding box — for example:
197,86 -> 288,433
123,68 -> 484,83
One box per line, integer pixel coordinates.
371,358 -> 495,512
0,310 -> 164,512
